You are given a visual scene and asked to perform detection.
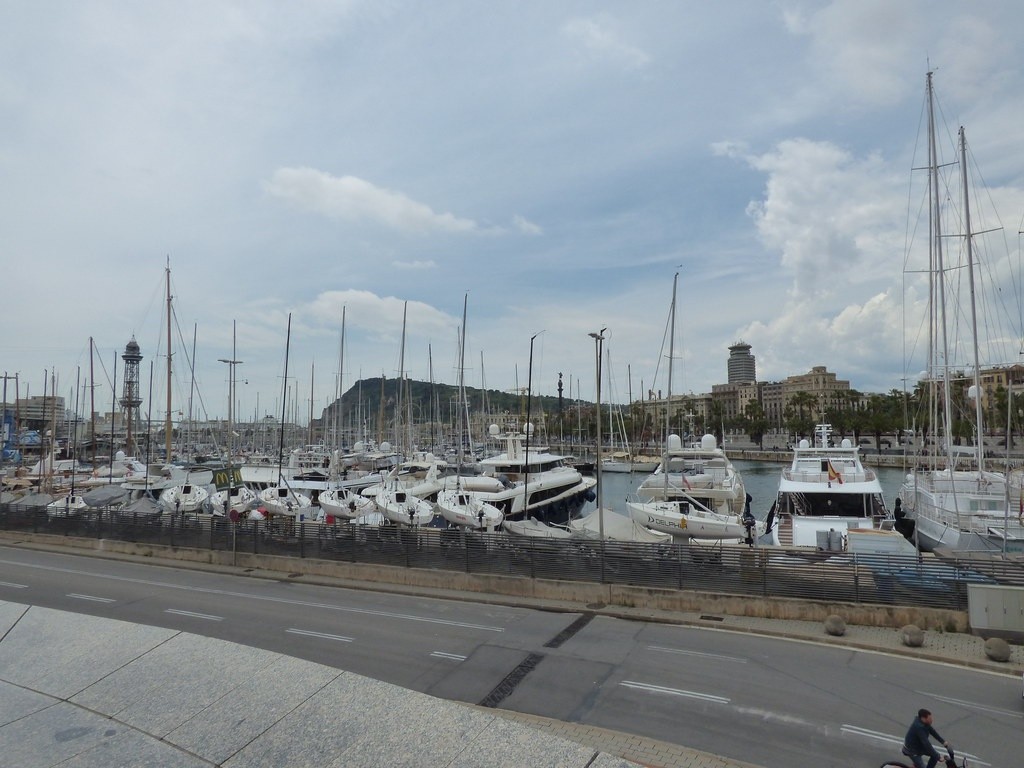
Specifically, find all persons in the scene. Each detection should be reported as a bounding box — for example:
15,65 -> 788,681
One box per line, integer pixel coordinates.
904,709 -> 948,768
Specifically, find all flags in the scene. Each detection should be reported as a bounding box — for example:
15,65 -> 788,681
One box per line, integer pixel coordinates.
827,460 -> 842,484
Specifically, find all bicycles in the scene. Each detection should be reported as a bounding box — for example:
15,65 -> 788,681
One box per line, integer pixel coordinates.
879,745 -> 970,768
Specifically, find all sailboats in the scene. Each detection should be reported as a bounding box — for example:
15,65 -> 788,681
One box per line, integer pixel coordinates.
0,59 -> 1024,575
